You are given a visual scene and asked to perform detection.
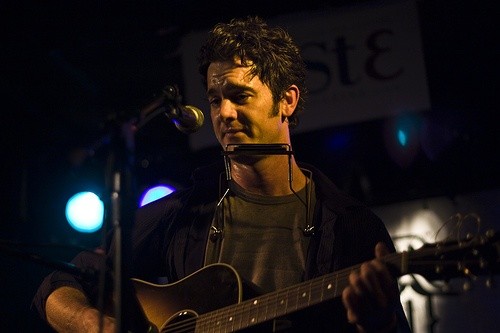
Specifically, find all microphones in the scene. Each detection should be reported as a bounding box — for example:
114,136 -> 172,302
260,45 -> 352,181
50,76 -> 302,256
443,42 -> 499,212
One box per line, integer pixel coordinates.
163,86 -> 203,133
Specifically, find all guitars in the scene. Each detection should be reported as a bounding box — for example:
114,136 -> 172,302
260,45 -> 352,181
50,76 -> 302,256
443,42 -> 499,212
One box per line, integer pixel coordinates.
92,228 -> 500,333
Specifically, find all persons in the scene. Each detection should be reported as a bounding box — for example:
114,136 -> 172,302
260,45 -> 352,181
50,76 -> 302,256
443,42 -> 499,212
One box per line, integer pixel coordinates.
29,15 -> 415,333
